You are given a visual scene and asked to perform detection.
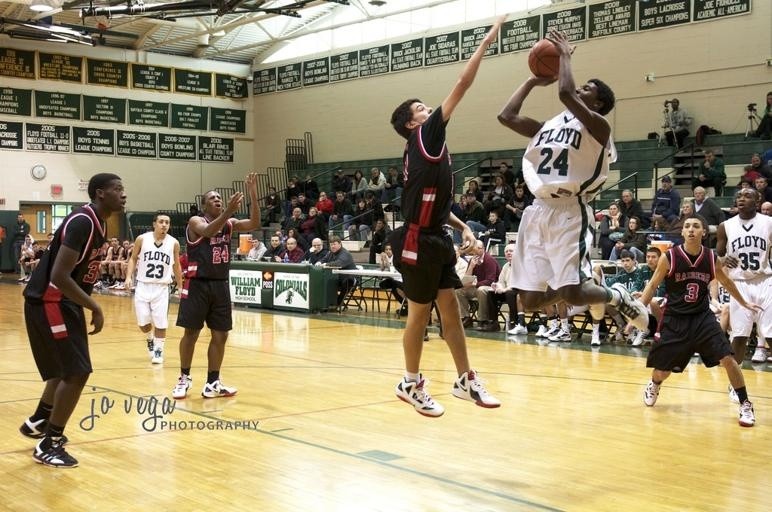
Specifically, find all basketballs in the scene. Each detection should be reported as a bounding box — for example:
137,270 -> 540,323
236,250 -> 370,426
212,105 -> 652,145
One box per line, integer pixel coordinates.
528,40 -> 560,80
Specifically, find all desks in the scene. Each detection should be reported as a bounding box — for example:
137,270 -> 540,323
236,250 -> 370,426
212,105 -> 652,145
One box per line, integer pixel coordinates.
227,259 -> 402,315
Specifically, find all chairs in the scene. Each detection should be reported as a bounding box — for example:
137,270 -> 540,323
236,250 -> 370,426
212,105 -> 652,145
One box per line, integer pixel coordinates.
466,267 -> 641,347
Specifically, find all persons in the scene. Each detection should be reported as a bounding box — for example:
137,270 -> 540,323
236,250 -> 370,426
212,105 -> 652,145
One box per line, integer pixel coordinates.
16,170 -> 129,471
497,27 -> 655,335
633,210 -> 768,430
385,13 -> 511,422
715,186 -> 772,404
11,148 -> 772,365
661,97 -> 694,150
756,91 -> 772,141
169,169 -> 265,400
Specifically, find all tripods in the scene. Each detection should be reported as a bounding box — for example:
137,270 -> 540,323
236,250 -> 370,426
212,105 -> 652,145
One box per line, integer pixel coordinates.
744,111 -> 763,141
658,107 -> 679,150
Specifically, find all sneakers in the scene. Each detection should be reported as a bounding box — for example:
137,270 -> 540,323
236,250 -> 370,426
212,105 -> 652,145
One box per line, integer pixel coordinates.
728,383 -> 739,403
644,380 -> 660,406
201,379 -> 237,398
152,349 -> 164,364
19,416 -> 68,444
582,276 -> 605,320
610,282 -> 649,331
172,375 -> 192,398
146,338 -> 156,356
94,280 -> 132,297
506,318 -> 769,364
32,435 -> 79,468
739,400 -> 755,426
395,373 -> 445,417
452,370 -> 501,408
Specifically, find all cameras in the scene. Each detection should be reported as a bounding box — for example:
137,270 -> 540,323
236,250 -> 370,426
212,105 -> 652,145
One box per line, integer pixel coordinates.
747,103 -> 756,111
665,99 -> 672,107
